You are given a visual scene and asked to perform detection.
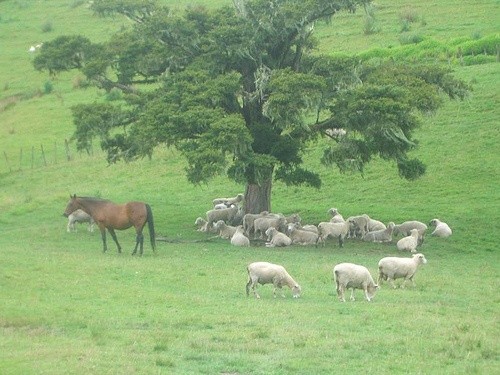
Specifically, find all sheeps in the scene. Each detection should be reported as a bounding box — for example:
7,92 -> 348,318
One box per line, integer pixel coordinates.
246,262 -> 301,300
429,218 -> 453,238
334,263 -> 379,302
377,253 -> 427,288
66,209 -> 94,233
196,193 -> 429,253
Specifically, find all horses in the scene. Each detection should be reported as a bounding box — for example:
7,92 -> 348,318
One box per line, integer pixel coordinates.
63,194 -> 156,256
